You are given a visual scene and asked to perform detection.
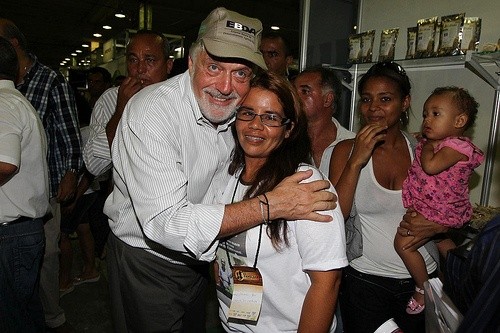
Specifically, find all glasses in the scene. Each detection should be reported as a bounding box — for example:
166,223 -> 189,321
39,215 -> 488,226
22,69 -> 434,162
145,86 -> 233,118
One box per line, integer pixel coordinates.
367,61 -> 406,76
234,106 -> 293,127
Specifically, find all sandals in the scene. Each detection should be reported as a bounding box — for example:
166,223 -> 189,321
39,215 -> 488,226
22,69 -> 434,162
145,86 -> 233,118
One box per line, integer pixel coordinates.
405,286 -> 426,315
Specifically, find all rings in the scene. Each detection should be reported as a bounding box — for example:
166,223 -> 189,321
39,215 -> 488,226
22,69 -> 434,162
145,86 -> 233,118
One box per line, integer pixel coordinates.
407,230 -> 410,236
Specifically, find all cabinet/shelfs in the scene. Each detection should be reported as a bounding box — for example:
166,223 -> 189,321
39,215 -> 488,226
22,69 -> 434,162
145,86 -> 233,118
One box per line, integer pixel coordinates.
300,1 -> 500,209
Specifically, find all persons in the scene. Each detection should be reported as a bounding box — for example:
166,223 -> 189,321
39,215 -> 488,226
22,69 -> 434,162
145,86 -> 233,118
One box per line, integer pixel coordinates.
0,18 -> 76,333
204,72 -> 349,333
394,87 -> 486,315
82,31 -> 175,285
257,30 -> 293,86
57,166 -> 101,298
292,67 -> 358,181
102,7 -> 337,333
433,216 -> 500,333
0,37 -> 52,333
330,58 -> 457,332
86,68 -> 114,112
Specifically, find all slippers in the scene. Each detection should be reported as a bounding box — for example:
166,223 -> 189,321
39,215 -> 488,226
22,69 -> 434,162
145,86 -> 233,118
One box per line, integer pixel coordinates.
73,271 -> 100,286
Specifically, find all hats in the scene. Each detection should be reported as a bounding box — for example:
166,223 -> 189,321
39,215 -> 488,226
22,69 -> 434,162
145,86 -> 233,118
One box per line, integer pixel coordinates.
195,7 -> 269,73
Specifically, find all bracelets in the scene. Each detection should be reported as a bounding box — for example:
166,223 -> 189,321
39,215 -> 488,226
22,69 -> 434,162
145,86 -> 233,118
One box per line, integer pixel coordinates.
257,194 -> 271,224
